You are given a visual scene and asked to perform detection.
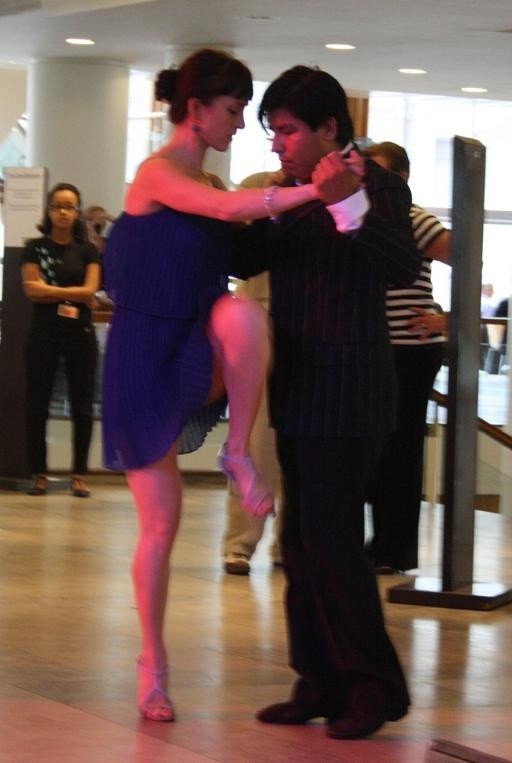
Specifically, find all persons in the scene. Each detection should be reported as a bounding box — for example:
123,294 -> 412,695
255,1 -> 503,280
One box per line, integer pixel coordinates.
480,281 -> 508,346
227,61 -> 415,741
15,180 -> 102,500
93,46 -> 369,726
81,201 -> 123,315
361,136 -> 457,580
219,164 -> 293,578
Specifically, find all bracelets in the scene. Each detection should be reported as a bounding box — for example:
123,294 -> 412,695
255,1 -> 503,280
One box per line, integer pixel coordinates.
261,183 -> 281,226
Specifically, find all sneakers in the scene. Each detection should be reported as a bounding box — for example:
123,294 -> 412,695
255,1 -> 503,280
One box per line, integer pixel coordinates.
223,550 -> 252,576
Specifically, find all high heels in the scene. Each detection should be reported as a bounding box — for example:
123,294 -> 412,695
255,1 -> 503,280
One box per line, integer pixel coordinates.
216,439 -> 275,520
133,653 -> 174,723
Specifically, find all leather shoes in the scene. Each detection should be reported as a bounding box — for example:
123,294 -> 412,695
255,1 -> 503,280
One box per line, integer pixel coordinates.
252,675 -> 410,739
27,475 -> 91,498
